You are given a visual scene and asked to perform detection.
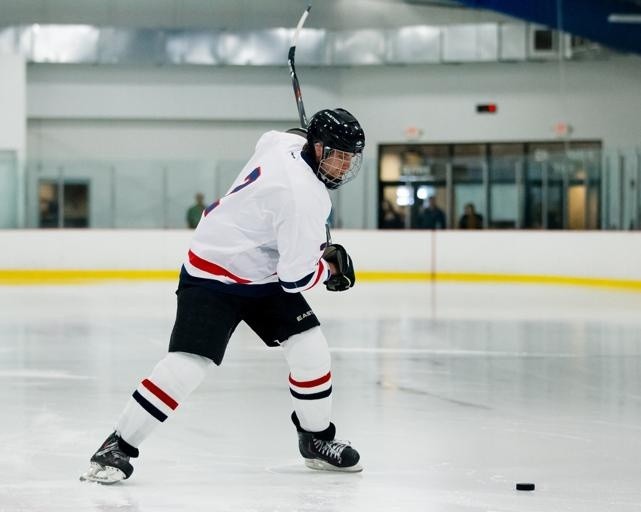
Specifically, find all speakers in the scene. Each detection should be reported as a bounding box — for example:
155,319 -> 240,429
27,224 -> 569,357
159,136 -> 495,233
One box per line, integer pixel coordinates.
90,430 -> 139,478
291,411 -> 360,468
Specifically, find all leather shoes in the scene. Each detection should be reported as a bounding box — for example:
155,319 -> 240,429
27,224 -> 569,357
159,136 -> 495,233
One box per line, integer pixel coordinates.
322,244 -> 355,291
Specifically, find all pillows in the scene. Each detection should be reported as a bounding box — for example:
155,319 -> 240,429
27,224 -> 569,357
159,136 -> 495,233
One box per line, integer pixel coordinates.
306,108 -> 365,154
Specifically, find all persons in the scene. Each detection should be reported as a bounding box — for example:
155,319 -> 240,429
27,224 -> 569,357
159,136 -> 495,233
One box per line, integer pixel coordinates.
456,203 -> 483,228
379,198 -> 404,230
414,195 -> 447,228
184,189 -> 206,230
86,105 -> 366,480
